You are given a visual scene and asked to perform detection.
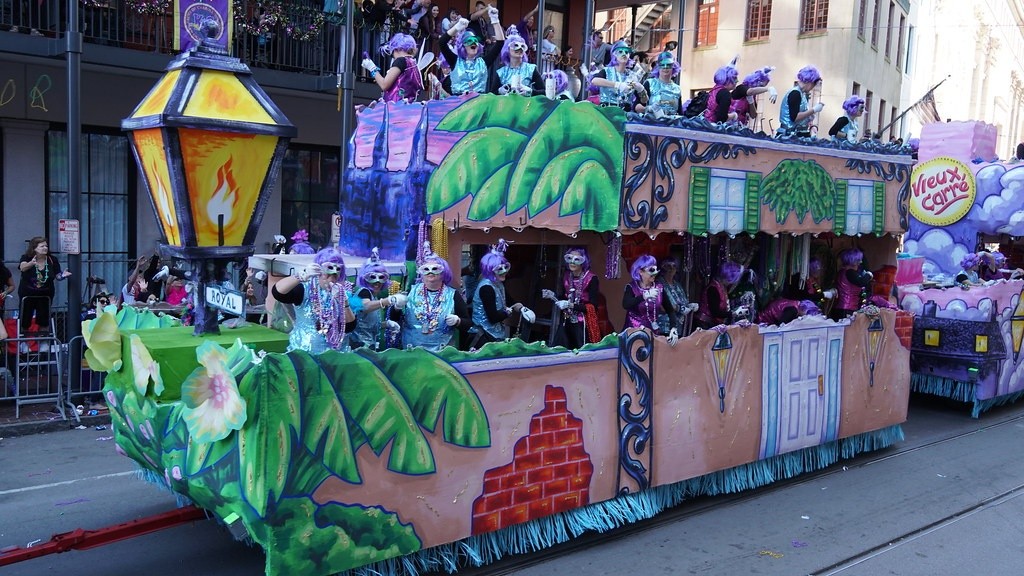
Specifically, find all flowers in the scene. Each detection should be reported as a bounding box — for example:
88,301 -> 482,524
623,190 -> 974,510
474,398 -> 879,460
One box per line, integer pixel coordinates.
82,0 -> 326,41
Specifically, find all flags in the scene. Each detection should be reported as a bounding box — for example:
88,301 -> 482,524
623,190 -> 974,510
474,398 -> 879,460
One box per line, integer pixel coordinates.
912,90 -> 942,125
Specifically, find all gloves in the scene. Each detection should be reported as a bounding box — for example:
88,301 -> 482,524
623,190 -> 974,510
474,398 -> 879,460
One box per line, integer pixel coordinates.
734,305 -> 750,317
388,293 -> 409,308
487,4 -> 500,24
296,262 -> 321,282
731,112 -> 738,121
643,287 -> 658,300
680,303 -> 699,315
521,306 -> 536,323
455,18 -> 470,31
824,291 -> 832,299
614,81 -> 644,93
445,315 -> 461,327
867,271 -> 873,276
543,288 -> 570,310
812,103 -> 824,114
667,327 -> 679,347
362,59 -> 377,71
386,320 -> 400,333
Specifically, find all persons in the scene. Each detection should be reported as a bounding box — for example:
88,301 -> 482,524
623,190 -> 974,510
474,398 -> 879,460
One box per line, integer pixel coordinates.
542,247 -> 599,348
792,256 -> 838,314
889,135 -> 904,147
461,243 -> 536,348
622,253 -> 699,348
7,0 -> 45,37
1017,141 -> 1024,160
390,255 -> 468,351
80,248 -> 188,410
757,298 -> 825,326
323,0 -> 864,144
695,261 -> 750,330
954,242 -> 1024,286
217,229 -> 356,355
0,237 -> 72,353
833,248 -> 873,322
353,247 -> 408,350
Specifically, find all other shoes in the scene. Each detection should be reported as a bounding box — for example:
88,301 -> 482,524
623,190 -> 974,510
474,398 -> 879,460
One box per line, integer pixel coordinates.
89,404 -> 108,410
19,342 -> 31,354
40,342 -> 49,352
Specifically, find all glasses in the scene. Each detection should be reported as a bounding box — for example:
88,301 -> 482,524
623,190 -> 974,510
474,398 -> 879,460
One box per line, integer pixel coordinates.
99,299 -> 110,305
172,278 -> 180,282
596,37 -> 604,39
549,30 -> 554,32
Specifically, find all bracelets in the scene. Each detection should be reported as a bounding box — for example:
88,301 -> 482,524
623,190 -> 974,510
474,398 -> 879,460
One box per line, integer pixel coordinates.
60,272 -> 66,279
379,298 -> 385,309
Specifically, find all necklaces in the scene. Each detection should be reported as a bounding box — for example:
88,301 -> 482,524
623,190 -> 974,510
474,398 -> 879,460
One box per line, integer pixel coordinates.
34,256 -> 49,283
861,287 -> 867,309
813,280 -> 825,302
645,274 -> 730,330
309,217 -> 622,350
